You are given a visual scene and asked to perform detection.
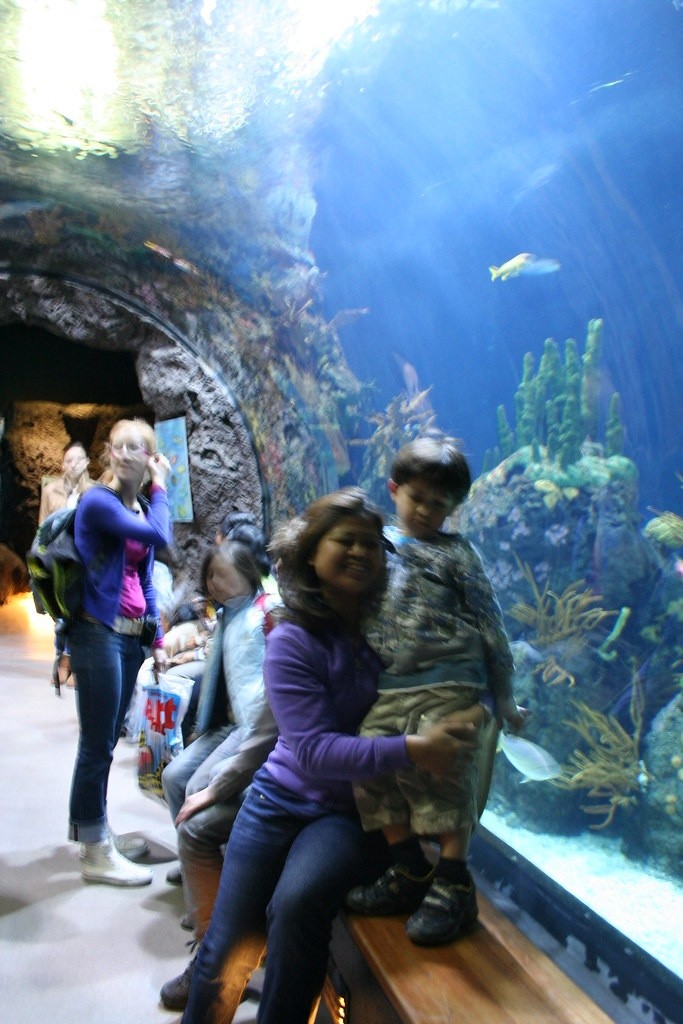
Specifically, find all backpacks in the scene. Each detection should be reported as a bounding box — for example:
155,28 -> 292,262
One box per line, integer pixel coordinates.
26,485 -> 151,626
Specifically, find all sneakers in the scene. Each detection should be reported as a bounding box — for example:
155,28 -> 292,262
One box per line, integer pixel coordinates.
346,863 -> 439,916
406,870 -> 478,945
79,826 -> 149,860
160,939 -> 198,1009
82,836 -> 154,886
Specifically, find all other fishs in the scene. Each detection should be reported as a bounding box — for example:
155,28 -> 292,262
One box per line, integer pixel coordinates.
496,640 -> 563,784
488,253 -> 561,282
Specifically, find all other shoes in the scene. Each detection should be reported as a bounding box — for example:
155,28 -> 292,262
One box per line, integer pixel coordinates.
165,866 -> 184,886
181,915 -> 193,929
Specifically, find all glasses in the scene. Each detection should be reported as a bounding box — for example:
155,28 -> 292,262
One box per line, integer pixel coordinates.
110,442 -> 153,458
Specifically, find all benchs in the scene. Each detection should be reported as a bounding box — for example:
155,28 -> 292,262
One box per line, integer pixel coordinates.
341,882 -> 615,1024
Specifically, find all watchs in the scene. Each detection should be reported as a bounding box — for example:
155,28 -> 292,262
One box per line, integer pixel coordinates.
479,701 -> 491,720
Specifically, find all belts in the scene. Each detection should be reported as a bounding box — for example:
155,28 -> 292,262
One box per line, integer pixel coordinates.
83,615 -> 144,635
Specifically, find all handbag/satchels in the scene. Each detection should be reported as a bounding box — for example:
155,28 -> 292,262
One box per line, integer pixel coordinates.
140,614 -> 160,647
128,668 -> 195,809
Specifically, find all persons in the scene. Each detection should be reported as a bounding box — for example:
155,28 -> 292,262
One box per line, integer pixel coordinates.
181,486 -> 495,1024
38,420 -> 530,1024
